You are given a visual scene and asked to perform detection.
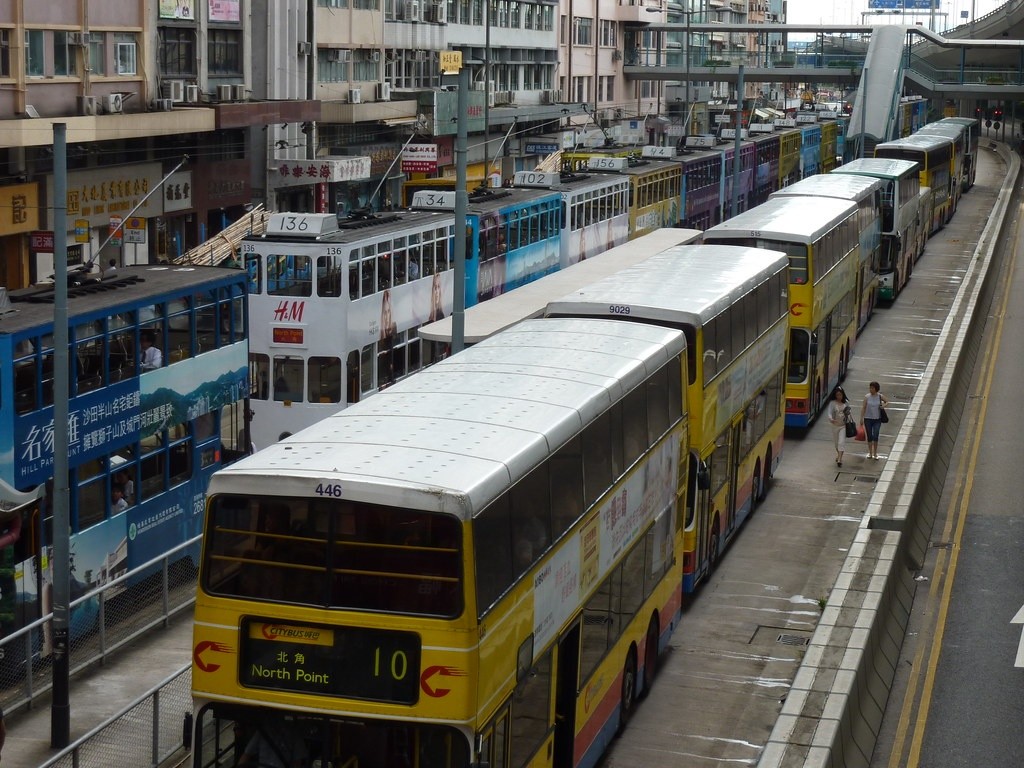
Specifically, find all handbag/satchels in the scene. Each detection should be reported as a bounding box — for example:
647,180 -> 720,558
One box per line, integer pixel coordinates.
878,394 -> 888,423
846,414 -> 857,437
855,424 -> 866,441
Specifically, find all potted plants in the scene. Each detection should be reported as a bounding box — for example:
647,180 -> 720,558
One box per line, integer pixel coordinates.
985,76 -> 1004,85
625,48 -> 638,66
703,60 -> 732,67
828,61 -> 856,69
774,60 -> 795,68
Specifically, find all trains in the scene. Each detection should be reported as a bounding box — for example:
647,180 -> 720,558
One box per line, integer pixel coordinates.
0,94 -> 931,693
179,115 -> 976,768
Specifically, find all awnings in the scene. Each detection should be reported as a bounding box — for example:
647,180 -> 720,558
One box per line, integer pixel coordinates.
754,108 -> 785,121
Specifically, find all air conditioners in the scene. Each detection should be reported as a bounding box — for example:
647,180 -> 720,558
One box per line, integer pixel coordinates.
386,52 -> 398,62
348,89 -> 360,103
601,108 -> 622,120
543,89 -> 562,103
184,85 -> 197,102
377,82 -> 390,100
404,1 -> 418,23
370,52 -> 379,62
338,50 -> 350,63
216,84 -> 245,100
299,42 -> 311,55
154,100 -> 172,112
163,80 -> 184,102
77,95 -> 97,116
430,4 -> 447,23
473,81 -> 494,107
416,51 -> 426,62
102,94 -> 122,113
771,90 -> 780,101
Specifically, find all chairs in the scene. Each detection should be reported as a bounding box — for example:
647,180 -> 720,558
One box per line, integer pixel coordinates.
13,329 -> 243,416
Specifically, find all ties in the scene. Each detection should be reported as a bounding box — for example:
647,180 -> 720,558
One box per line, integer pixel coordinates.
142,351 -> 145,364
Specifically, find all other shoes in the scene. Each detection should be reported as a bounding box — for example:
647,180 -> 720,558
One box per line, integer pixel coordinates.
837,462 -> 842,467
874,455 -> 879,460
867,454 -> 872,458
836,457 -> 838,462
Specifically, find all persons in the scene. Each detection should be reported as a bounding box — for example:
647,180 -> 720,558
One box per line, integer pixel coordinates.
429,274 -> 445,322
402,251 -> 418,282
381,290 -> 397,340
30,536 -> 99,658
111,469 -> 133,515
828,386 -> 851,467
578,228 -> 587,262
107,259 -> 116,270
140,330 -> 162,373
606,219 -> 615,251
498,498 -> 546,567
860,382 -> 889,460
629,43 -> 642,64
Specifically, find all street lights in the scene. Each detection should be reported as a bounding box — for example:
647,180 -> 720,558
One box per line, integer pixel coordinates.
645,6 -> 732,137
